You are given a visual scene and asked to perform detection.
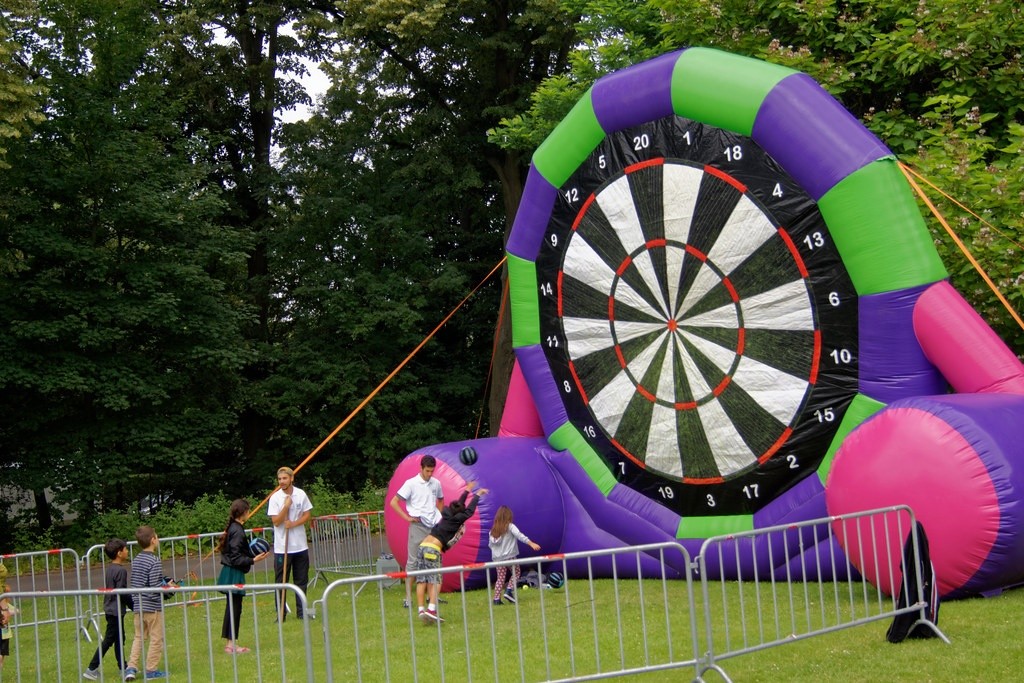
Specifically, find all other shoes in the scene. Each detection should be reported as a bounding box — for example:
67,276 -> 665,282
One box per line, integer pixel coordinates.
493,600 -> 504,605
83,668 -> 100,681
426,598 -> 448,604
146,670 -> 172,680
424,609 -> 445,622
404,601 -> 413,607
124,668 -> 136,681
418,610 -> 428,619
503,591 -> 515,603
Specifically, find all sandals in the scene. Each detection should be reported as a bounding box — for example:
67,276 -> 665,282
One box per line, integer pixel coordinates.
227,646 -> 249,653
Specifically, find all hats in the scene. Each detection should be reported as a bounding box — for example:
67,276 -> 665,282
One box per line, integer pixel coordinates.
277,467 -> 293,475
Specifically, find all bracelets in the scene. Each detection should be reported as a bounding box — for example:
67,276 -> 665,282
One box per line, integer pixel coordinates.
2,606 -> 9,611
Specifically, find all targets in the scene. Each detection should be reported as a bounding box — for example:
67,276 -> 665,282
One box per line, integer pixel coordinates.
538,113 -> 861,520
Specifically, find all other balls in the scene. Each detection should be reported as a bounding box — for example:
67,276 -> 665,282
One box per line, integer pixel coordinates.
548,572 -> 563,589
249,537 -> 270,557
459,445 -> 477,465
162,576 -> 177,599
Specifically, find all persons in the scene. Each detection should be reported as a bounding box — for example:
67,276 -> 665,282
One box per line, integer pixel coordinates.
488,505 -> 540,606
0,564 -> 19,670
415,480 -> 490,625
124,526 -> 176,681
390,454 -> 448,608
83,538 -> 134,681
216,497 -> 270,653
266,468 -> 313,623
